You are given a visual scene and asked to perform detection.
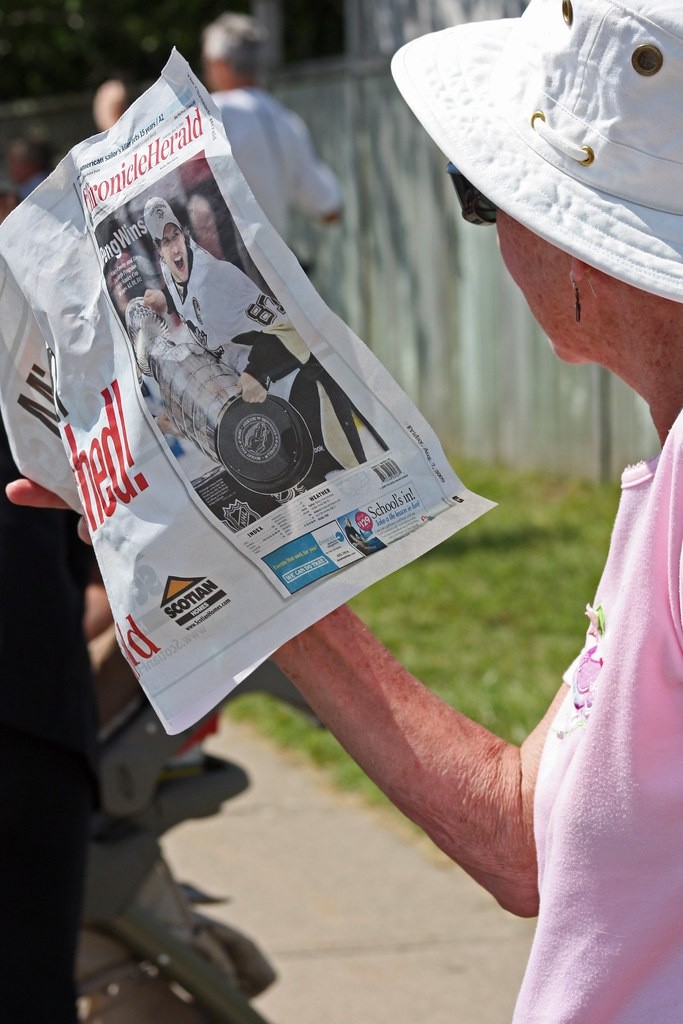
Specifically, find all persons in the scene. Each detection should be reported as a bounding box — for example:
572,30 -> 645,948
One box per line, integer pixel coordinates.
143,197 -> 309,406
1,16 -> 347,1024
4,1 -> 682,1021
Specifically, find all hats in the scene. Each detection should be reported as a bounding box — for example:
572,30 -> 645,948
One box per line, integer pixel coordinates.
203,12 -> 267,60
144,196 -> 182,241
391,0 -> 683,305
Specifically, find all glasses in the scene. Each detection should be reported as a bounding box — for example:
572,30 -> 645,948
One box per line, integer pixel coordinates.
445,161 -> 497,227
